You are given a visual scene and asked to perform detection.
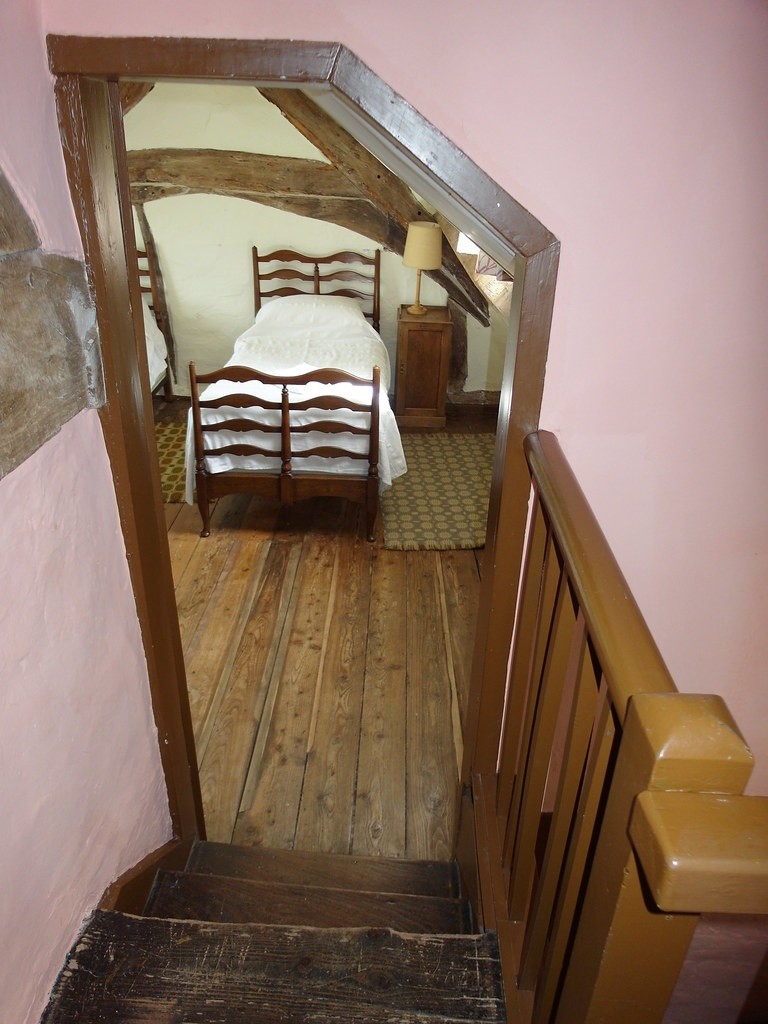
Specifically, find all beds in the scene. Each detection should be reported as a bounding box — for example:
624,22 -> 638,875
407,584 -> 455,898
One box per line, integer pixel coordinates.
184,247 -> 407,543
135,243 -> 173,404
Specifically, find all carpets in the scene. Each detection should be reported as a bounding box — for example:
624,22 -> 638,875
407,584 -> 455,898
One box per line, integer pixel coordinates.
151,419 -> 216,504
377,433 -> 493,552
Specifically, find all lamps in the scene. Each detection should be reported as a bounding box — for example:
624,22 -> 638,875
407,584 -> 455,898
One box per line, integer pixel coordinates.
402,221 -> 442,315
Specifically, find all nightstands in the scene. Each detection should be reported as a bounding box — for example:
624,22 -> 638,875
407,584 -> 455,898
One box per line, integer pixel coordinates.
394,304 -> 452,433
